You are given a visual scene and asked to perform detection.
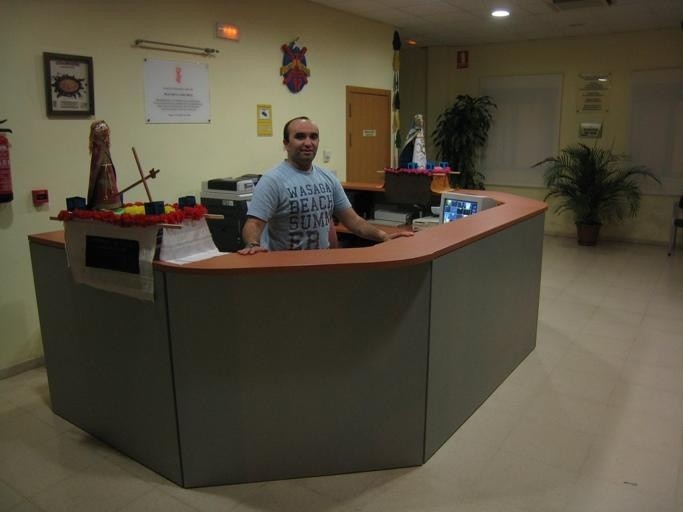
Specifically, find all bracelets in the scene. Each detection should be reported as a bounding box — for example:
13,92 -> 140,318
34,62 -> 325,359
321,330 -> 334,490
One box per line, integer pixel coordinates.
245,243 -> 260,247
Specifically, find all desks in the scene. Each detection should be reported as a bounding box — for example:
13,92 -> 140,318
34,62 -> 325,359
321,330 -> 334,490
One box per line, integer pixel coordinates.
334,217 -> 417,241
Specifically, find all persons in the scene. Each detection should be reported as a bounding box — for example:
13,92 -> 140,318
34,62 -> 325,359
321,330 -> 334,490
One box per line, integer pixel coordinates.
237,116 -> 417,255
402,114 -> 427,168
87,121 -> 123,209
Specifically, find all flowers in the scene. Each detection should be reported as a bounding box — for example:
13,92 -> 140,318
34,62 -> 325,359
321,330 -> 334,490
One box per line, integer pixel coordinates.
59,202 -> 207,226
386,165 -> 449,176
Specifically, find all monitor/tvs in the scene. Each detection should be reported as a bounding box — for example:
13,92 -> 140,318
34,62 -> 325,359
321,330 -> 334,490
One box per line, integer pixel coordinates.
439,192 -> 495,224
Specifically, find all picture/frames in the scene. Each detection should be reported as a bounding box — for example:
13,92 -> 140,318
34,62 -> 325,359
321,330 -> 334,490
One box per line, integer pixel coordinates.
44,52 -> 95,116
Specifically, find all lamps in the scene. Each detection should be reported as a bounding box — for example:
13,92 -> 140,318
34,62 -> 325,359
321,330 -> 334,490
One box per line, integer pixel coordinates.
216,23 -> 239,40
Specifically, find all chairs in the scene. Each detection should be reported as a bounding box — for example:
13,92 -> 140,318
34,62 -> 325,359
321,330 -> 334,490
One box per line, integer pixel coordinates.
668,196 -> 683,255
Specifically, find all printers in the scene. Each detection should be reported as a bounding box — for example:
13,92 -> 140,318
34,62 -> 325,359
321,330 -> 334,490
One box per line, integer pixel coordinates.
201,179 -> 255,200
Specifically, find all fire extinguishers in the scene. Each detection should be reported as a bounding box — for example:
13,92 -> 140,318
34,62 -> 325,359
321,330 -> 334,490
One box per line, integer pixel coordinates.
0,119 -> 13,203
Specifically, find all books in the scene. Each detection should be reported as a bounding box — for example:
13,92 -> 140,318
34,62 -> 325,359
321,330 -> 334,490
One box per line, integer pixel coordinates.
199,173 -> 261,199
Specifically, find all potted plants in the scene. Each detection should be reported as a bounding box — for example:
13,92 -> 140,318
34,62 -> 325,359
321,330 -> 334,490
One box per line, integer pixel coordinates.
532,141 -> 662,246
